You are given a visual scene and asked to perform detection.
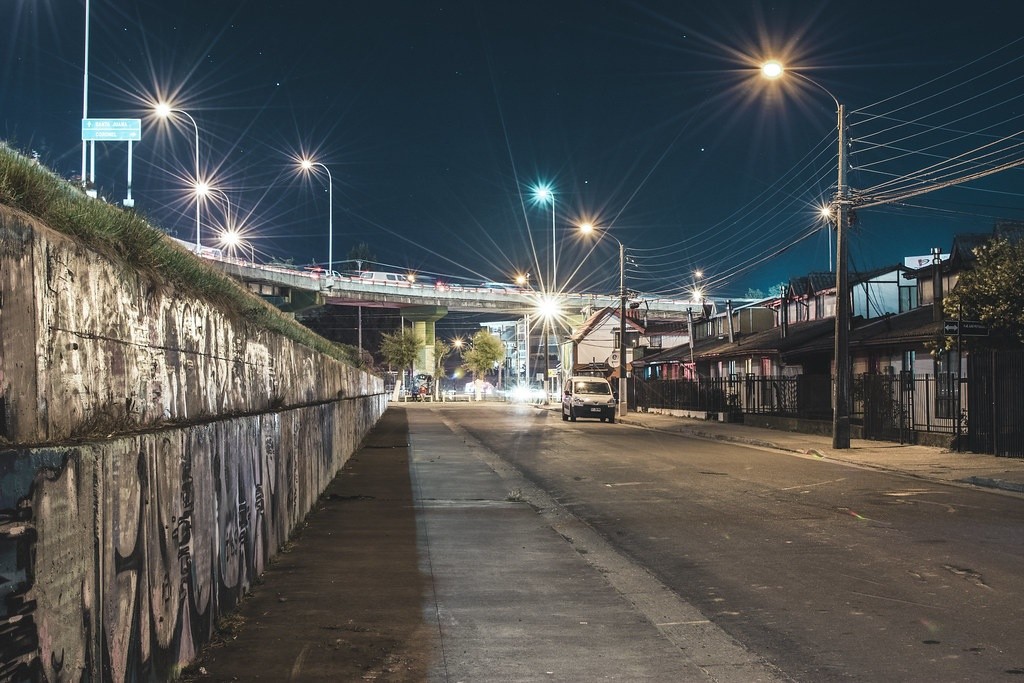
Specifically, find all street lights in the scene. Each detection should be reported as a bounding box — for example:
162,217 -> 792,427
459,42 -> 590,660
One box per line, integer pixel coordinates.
195,181 -> 232,256
301,159 -> 333,276
155,102 -> 202,254
762,59 -> 851,449
455,339 -> 474,383
538,298 -> 560,405
534,185 -> 558,293
580,220 -> 628,416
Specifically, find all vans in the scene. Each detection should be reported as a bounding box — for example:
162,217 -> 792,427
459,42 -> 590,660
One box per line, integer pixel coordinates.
361,271 -> 412,285
561,375 -> 616,423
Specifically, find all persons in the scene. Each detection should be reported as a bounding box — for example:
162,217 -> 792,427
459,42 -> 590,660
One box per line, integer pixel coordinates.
419,385 -> 426,403
412,386 -> 419,402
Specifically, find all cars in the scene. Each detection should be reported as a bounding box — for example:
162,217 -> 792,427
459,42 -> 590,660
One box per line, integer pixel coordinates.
303,267 -> 341,278
